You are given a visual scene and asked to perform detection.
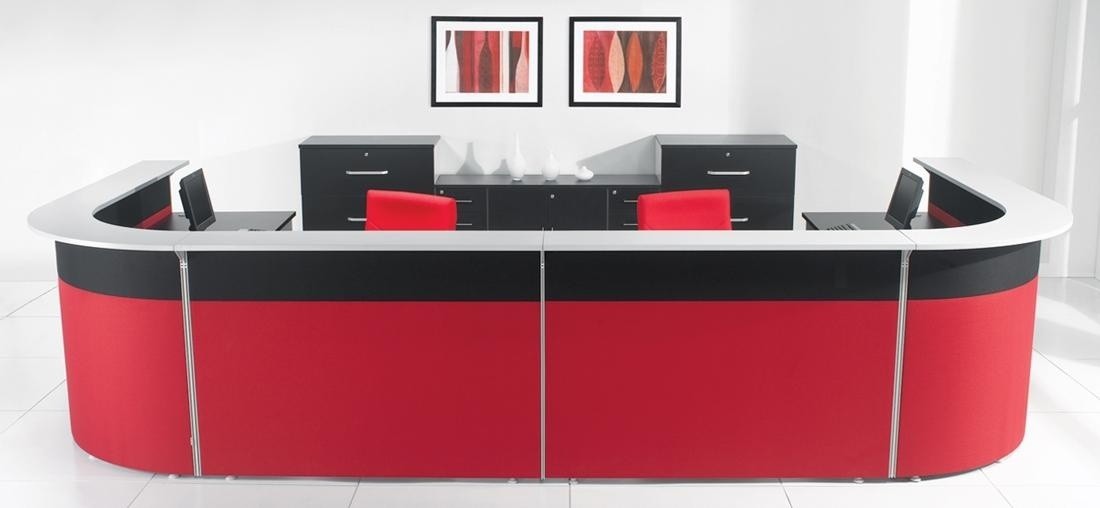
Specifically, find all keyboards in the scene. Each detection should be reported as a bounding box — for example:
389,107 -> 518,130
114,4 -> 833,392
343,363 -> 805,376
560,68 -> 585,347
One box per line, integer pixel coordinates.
826,222 -> 860,231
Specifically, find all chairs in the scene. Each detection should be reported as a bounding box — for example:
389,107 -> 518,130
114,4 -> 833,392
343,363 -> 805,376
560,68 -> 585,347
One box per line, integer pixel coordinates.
365,189 -> 458,229
634,189 -> 730,228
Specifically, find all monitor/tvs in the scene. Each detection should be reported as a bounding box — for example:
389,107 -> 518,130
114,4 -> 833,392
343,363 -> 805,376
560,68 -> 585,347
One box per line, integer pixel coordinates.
178,167 -> 216,232
883,167 -> 925,230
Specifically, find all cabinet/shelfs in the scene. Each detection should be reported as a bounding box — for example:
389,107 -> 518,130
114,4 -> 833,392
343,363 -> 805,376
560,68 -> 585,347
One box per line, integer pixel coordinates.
436,188 -> 487,228
609,188 -> 663,229
654,134 -> 799,230
299,134 -> 442,231
489,188 -> 607,229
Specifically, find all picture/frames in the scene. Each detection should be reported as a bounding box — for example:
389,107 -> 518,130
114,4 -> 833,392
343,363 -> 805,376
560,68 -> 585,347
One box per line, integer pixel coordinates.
567,14 -> 683,110
430,14 -> 544,108
